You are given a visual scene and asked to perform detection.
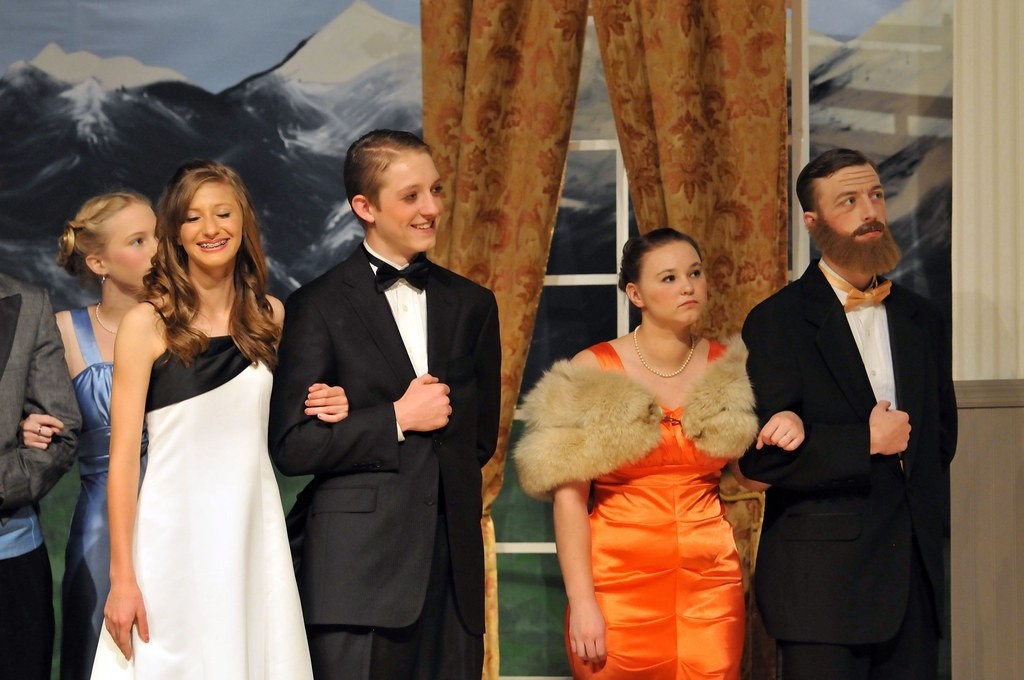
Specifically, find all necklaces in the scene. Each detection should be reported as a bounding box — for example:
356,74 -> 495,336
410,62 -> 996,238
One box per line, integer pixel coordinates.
95,301 -> 117,334
634,325 -> 694,378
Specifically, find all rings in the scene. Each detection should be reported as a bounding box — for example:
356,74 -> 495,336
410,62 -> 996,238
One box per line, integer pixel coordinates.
38,425 -> 42,436
787,434 -> 792,439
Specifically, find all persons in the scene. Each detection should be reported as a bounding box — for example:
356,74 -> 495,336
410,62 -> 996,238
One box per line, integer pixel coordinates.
0,273 -> 83,680
91,161 -> 350,680
266,130 -> 502,680
739,146 -> 959,680
17,188 -> 159,643
514,226 -> 805,680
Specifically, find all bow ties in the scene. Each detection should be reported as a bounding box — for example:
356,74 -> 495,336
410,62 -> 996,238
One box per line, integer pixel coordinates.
362,243 -> 430,294
818,263 -> 890,313
